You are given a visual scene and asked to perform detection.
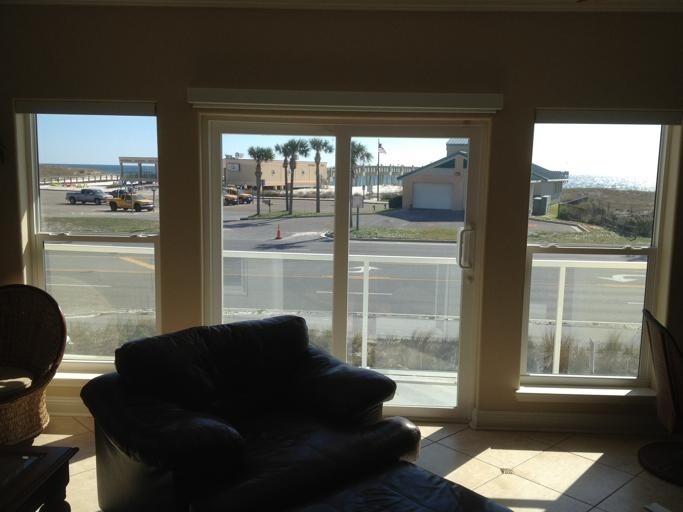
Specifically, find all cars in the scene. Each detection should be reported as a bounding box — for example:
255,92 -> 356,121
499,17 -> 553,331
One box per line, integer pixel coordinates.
222,185 -> 254,206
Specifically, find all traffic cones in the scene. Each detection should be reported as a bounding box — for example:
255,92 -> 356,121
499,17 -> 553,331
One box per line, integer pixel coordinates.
275,224 -> 283,239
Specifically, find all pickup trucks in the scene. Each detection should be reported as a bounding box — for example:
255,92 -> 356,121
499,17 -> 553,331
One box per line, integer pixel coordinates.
66,189 -> 156,212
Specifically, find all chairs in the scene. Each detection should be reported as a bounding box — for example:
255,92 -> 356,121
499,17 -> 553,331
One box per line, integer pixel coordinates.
637,310 -> 682,484
79,311 -> 421,511
0,283 -> 67,447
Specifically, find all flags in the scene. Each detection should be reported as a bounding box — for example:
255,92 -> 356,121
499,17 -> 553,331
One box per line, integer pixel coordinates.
378,140 -> 387,153
